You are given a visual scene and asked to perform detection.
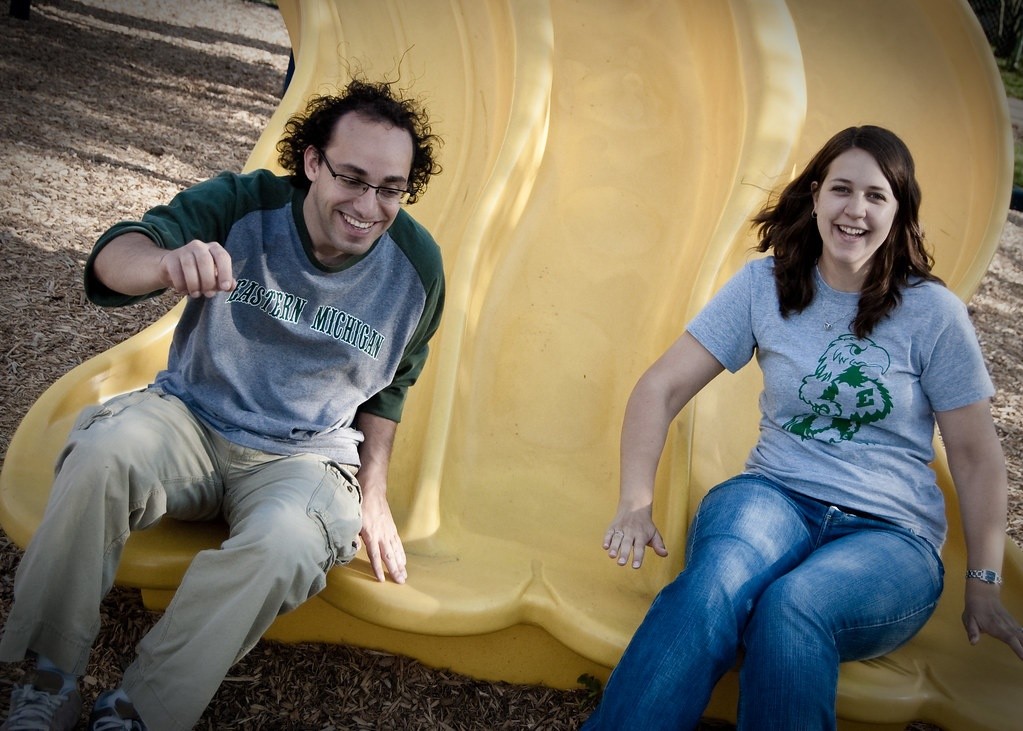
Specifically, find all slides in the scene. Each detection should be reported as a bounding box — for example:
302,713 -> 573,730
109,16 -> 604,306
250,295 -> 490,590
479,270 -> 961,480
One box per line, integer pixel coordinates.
0,0 -> 1023,731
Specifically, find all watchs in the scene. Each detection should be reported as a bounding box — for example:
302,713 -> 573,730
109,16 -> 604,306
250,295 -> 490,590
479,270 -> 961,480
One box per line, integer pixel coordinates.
964,569 -> 1003,585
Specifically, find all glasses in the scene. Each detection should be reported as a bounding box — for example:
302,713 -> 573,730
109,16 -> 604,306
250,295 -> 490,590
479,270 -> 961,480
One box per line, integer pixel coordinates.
317,151 -> 412,206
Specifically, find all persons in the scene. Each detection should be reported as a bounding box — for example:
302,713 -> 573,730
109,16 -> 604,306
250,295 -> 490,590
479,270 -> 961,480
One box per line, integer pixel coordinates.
582,122 -> 1013,731
0,67 -> 451,731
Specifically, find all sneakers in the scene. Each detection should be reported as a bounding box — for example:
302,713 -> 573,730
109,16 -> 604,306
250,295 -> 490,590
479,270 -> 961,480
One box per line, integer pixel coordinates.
2,663 -> 80,731
92,690 -> 142,731
